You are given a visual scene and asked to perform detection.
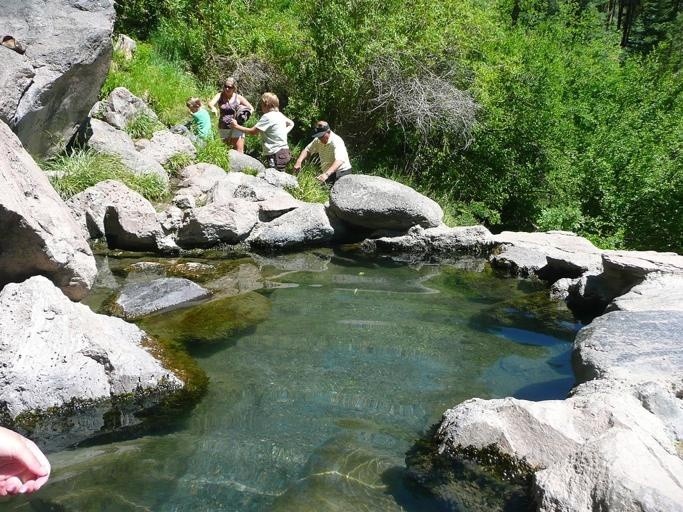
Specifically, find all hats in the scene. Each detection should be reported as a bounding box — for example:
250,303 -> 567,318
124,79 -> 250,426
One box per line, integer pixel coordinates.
312,121 -> 331,138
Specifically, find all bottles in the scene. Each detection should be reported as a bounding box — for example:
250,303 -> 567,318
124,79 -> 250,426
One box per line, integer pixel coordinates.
268,156 -> 275,168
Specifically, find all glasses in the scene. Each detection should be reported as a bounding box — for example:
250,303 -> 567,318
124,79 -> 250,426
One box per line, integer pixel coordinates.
224,84 -> 233,89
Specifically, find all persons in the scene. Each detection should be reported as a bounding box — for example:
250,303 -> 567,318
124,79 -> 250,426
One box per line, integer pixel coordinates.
208,77 -> 254,154
185,96 -> 211,141
0,425 -> 51,497
228,92 -> 294,171
291,121 -> 352,184
